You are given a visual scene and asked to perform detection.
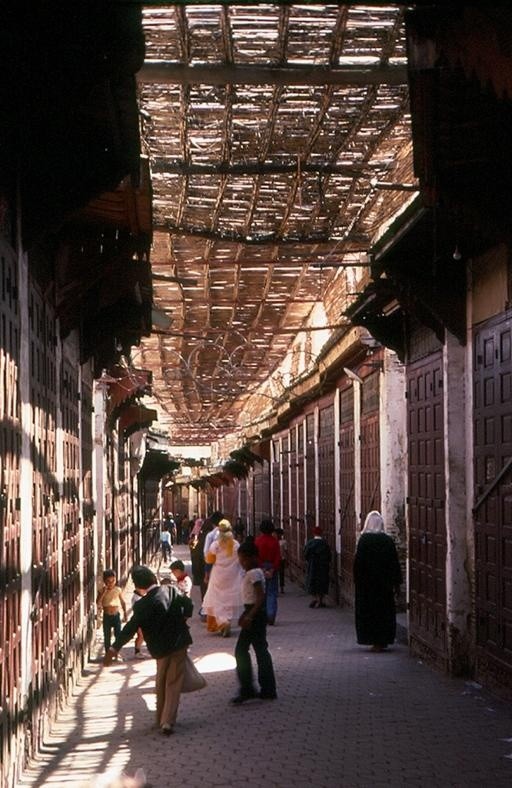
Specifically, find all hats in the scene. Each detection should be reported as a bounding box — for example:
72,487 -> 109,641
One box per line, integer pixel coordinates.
210,511 -> 225,525
258,520 -> 274,532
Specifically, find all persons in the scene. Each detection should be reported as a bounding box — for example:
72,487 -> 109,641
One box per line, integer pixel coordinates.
97,558 -> 192,737
353,510 -> 403,653
159,512 -> 197,564
190,509 -> 288,704
302,527 -> 332,608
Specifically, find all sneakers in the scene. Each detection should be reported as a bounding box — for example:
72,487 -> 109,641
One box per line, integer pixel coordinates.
257,692 -> 278,699
372,646 -> 384,652
231,690 -> 256,703
163,725 -> 173,734
135,653 -> 145,659
309,600 -> 326,608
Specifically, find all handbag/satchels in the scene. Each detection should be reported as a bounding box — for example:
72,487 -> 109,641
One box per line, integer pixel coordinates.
181,653 -> 206,693
